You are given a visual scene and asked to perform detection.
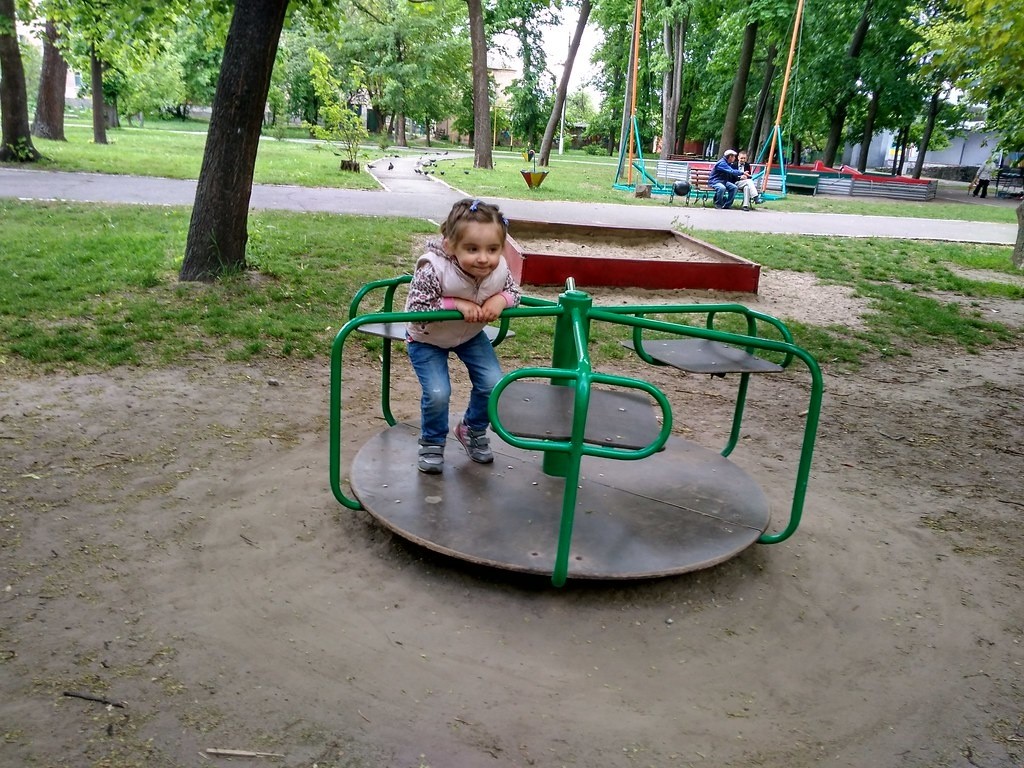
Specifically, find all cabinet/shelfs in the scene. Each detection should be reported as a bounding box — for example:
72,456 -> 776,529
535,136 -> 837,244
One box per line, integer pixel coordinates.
785,173 -> 820,196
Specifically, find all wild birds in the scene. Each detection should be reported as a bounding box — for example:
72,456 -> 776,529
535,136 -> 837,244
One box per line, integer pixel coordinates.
493,161 -> 498,168
332,150 -> 471,175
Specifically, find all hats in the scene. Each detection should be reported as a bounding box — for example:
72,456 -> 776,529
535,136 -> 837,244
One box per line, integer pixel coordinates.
724,149 -> 737,157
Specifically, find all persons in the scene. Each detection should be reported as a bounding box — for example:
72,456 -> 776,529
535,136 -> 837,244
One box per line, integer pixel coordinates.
728,151 -> 764,211
972,154 -> 996,199
708,149 -> 751,209
405,199 -> 521,475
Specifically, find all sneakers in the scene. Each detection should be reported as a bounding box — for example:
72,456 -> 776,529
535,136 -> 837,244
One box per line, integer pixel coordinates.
453,419 -> 494,463
417,433 -> 444,474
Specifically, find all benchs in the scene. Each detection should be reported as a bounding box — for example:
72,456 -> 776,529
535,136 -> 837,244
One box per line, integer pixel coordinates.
689,169 -> 760,209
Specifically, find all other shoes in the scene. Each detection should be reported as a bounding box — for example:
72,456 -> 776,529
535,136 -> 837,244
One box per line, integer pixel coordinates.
741,204 -> 749,211
754,199 -> 764,205
980,195 -> 986,198
715,203 -> 729,209
973,193 -> 978,197
